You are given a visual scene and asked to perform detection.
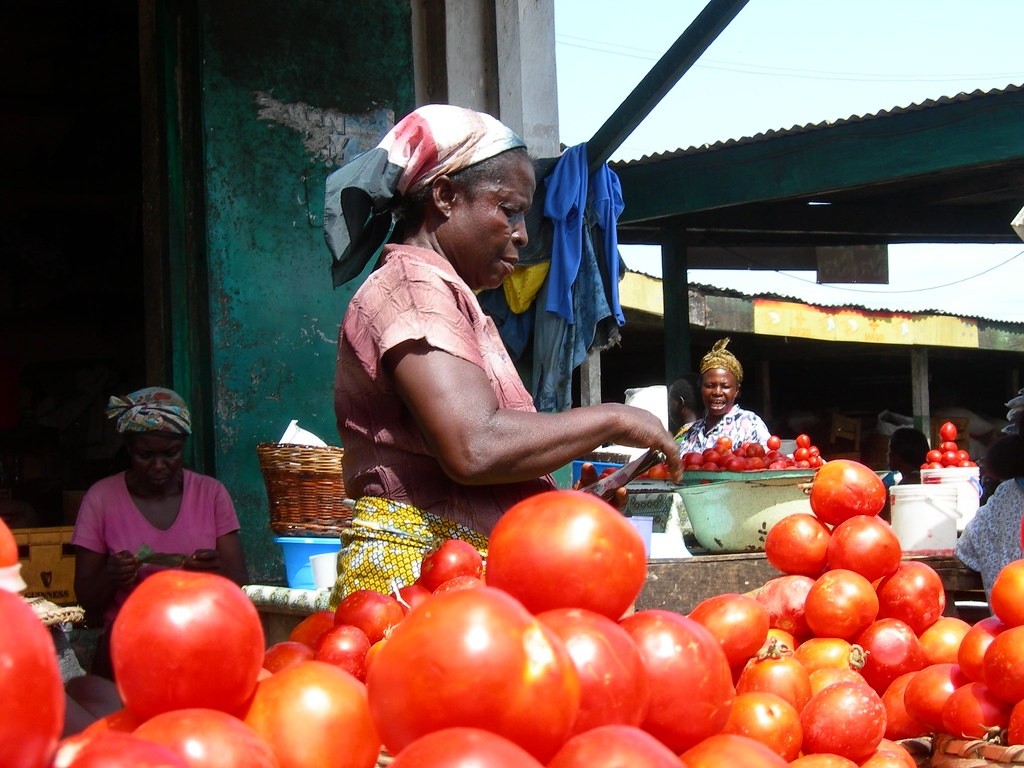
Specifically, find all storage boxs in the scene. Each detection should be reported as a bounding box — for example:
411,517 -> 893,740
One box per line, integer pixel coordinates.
11,526 -> 78,607
572,461 -> 625,486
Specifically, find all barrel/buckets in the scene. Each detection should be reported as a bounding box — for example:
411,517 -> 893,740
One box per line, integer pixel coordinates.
889,484 -> 958,558
919,466 -> 980,532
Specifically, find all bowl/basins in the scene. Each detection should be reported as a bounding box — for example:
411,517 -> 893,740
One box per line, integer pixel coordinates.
623,477 -> 674,533
273,537 -> 342,590
573,461 -> 625,490
673,471 -> 834,554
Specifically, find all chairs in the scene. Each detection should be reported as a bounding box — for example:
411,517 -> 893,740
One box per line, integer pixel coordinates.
824,412 -> 863,461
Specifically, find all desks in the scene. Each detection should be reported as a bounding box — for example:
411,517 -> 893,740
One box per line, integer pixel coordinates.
637,552 -> 983,617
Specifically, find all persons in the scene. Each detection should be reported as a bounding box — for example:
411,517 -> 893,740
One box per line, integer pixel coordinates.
668,379 -> 697,443
72,386 -> 250,682
881,428 -> 931,520
955,389 -> 1024,612
324,105 -> 681,611
678,338 -> 771,458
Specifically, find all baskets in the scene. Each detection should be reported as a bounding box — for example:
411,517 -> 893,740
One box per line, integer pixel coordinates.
256,444 -> 352,537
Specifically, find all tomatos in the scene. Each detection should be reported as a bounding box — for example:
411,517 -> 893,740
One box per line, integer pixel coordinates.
920,421 -> 978,469
0,457 -> 1024,768
598,433 -> 823,480
